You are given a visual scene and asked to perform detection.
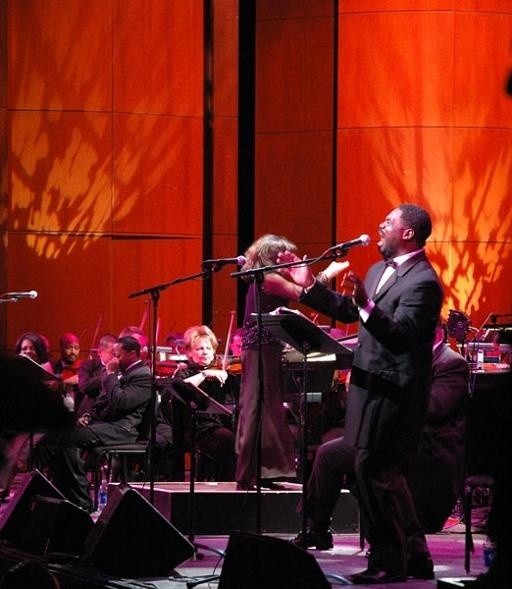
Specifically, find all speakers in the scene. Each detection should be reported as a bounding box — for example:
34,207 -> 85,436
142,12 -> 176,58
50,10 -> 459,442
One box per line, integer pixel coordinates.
82,482 -> 195,579
27,496 -> 93,556
217,528 -> 330,588
0,467 -> 65,550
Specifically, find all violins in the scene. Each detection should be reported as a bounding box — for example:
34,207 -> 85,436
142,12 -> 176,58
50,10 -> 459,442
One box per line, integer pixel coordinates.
61,353 -> 243,382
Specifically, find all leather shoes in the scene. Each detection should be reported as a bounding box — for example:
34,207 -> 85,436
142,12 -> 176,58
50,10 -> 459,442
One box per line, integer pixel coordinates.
288,529 -> 332,550
349,559 -> 434,583
238,483 -> 283,490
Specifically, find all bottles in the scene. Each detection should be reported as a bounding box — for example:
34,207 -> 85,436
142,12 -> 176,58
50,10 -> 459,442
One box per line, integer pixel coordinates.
88,484 -> 97,512
483,536 -> 495,573
97,480 -> 108,510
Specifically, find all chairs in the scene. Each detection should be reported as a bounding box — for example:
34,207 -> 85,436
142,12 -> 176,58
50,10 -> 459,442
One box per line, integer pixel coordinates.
84,383 -> 158,514
460,462 -> 496,576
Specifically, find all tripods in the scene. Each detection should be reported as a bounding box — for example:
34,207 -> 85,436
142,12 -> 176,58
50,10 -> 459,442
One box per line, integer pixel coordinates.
187,400 -> 226,558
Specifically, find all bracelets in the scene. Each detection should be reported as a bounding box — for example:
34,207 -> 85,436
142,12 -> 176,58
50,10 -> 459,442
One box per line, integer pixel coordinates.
318,270 -> 328,286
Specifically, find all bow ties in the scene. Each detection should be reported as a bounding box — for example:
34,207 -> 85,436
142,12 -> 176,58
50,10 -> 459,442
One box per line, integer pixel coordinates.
385,260 -> 395,267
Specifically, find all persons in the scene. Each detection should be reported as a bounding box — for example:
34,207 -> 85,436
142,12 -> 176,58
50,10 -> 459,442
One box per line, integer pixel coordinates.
234,234 -> 349,500
288,311 -> 472,554
167,335 -> 177,353
68,336 -> 152,510
156,324 -> 241,482
278,204 -> 444,581
16,327 -> 118,418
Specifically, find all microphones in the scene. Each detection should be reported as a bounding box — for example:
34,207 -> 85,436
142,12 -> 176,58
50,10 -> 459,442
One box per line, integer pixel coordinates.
4,290 -> 38,299
331,234 -> 370,250
205,256 -> 246,265
